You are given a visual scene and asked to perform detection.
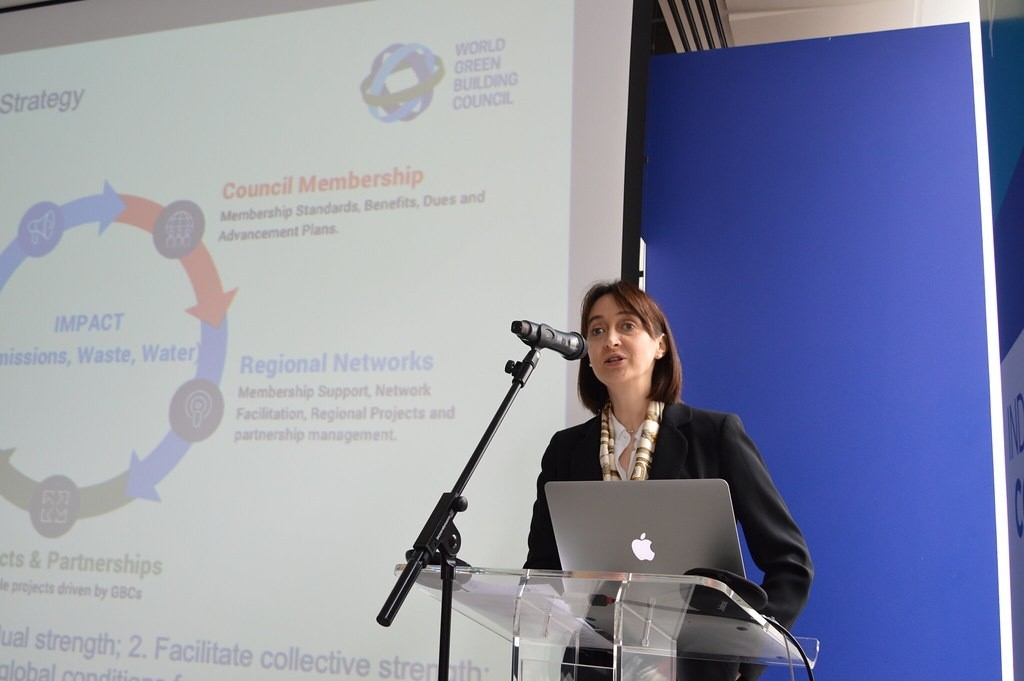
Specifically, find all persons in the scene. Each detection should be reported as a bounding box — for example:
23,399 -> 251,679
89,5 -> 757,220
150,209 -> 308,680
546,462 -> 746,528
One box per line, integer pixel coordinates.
526,280 -> 816,680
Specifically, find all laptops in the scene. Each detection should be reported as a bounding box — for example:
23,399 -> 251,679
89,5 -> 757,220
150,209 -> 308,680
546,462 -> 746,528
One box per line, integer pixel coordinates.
544,478 -> 746,611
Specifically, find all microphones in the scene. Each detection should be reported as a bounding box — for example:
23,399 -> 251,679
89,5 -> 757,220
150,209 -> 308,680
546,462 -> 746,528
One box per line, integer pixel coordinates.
511,320 -> 589,362
